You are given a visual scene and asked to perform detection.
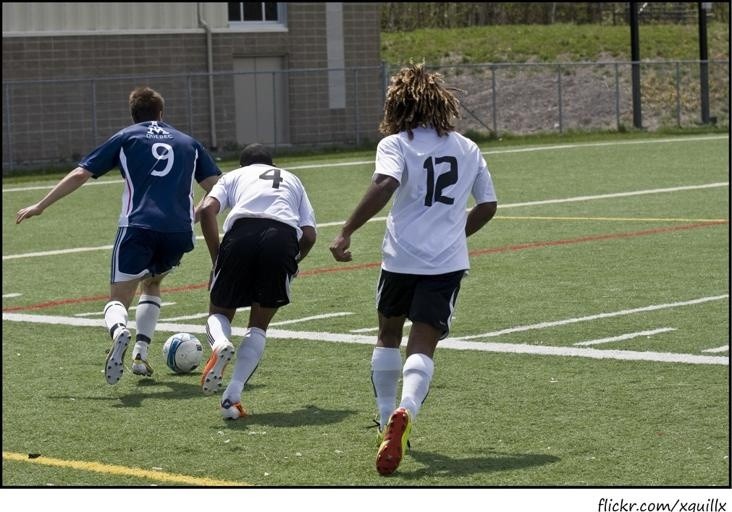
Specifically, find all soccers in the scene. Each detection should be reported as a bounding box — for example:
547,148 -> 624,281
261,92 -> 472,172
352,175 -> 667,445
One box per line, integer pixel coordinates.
162,333 -> 202,372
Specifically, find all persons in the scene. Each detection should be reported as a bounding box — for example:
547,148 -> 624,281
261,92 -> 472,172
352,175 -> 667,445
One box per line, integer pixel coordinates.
16,88 -> 225,384
329,62 -> 497,473
201,144 -> 317,419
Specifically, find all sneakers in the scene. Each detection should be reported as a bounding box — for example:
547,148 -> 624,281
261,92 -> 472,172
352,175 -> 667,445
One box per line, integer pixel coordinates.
376,408 -> 411,475
202,342 -> 234,396
221,400 -> 246,419
376,424 -> 389,448
131,352 -> 154,376
105,328 -> 131,384
